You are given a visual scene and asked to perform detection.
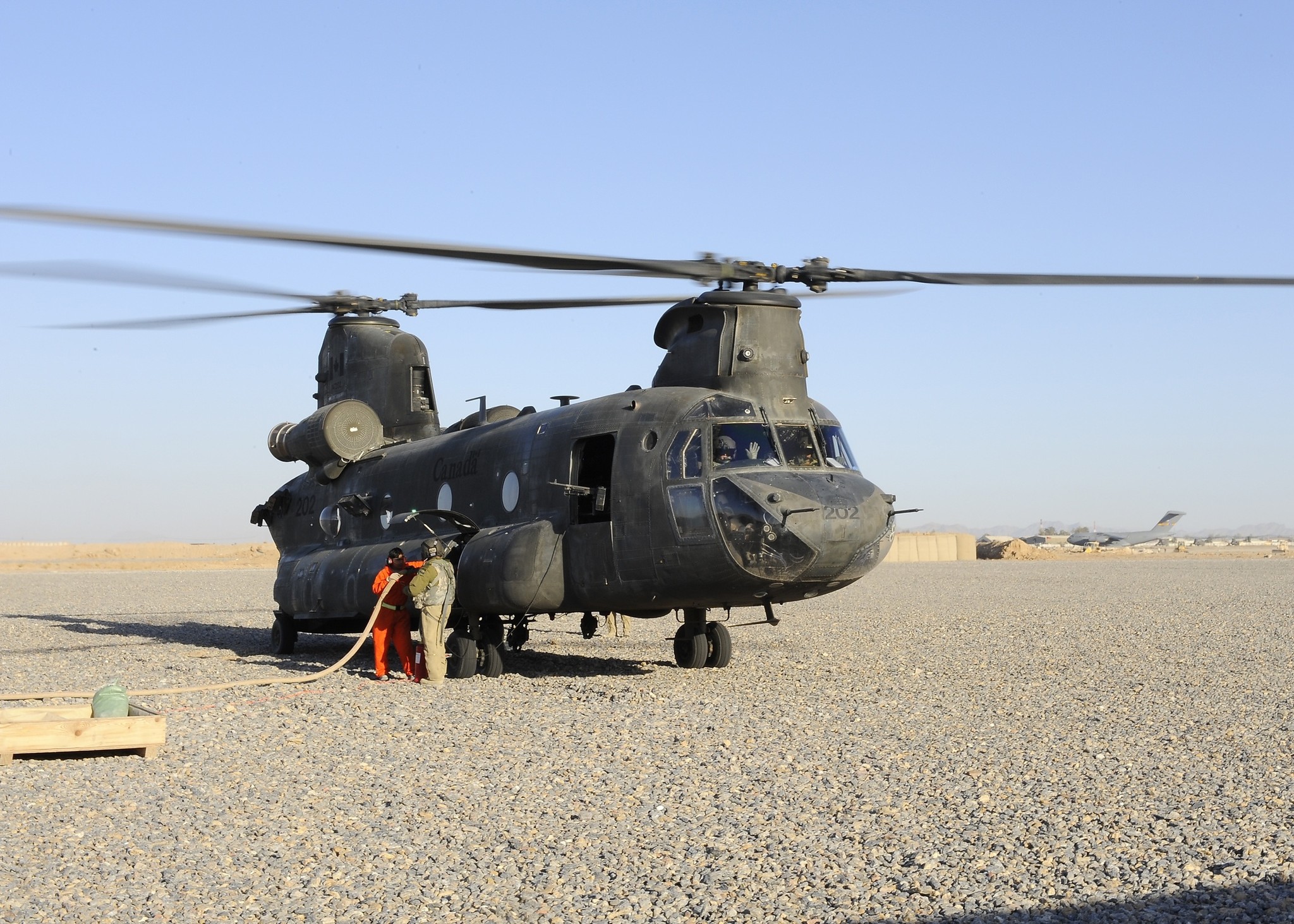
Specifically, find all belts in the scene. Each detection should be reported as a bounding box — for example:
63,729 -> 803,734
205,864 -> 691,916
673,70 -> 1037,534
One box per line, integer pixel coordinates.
381,603 -> 405,610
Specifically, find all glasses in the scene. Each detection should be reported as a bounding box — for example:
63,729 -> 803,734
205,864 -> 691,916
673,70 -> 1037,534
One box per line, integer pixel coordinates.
392,557 -> 407,564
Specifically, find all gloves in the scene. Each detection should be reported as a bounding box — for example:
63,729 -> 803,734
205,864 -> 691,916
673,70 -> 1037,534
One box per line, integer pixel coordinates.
386,573 -> 400,581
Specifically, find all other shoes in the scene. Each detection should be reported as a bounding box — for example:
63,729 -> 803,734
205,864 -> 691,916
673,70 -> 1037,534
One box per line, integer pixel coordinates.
380,675 -> 388,681
408,675 -> 415,680
420,678 -> 444,688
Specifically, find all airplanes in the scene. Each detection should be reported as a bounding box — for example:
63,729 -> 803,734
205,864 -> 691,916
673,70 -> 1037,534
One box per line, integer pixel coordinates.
1066,510 -> 1186,549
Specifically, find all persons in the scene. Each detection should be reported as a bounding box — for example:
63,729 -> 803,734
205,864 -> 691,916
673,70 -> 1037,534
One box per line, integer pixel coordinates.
712,436 -> 762,470
402,538 -> 456,688
371,549 -> 426,682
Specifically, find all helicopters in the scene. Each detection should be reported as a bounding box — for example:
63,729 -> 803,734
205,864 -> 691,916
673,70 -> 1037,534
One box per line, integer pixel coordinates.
1,204 -> 1294,681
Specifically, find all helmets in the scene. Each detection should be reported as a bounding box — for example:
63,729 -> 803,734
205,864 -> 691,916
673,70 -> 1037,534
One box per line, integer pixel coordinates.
421,540 -> 444,560
